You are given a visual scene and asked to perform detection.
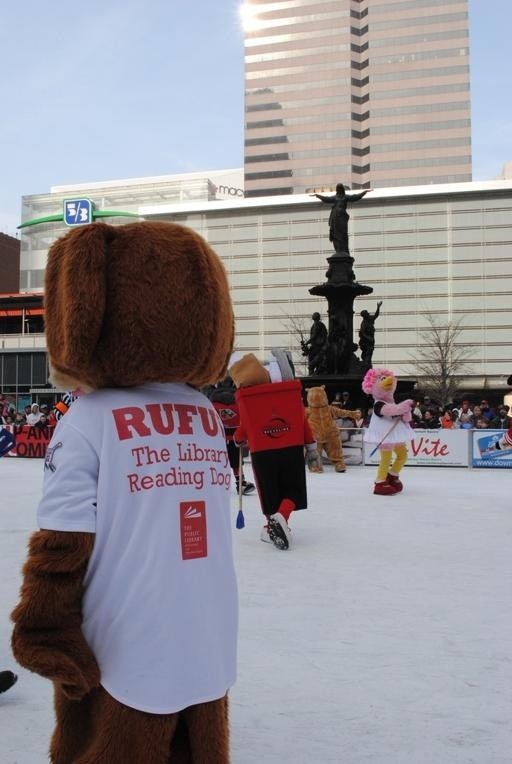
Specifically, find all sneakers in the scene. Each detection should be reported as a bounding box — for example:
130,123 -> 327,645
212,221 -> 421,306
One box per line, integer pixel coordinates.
0,670 -> 18,693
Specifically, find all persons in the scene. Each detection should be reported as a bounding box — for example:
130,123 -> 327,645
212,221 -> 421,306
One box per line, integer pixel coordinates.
301,312 -> 327,375
328,390 -> 512,431
359,299 -> 383,371
1,392 -> 58,433
308,182 -> 374,257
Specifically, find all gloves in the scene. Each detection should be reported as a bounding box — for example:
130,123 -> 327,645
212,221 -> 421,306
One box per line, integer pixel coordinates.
305,440 -> 321,472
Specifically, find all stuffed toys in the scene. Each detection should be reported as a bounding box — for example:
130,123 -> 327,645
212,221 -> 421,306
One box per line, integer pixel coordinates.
303,383 -> 365,474
228,346 -> 316,552
6,220 -> 240,762
360,367 -> 415,497
